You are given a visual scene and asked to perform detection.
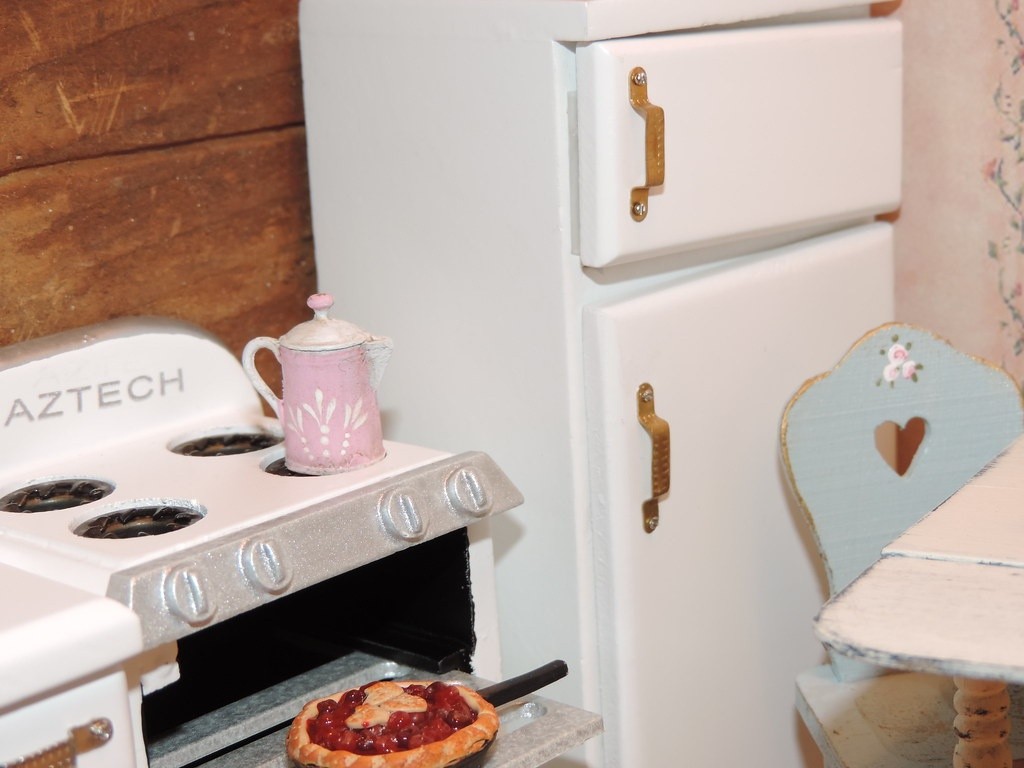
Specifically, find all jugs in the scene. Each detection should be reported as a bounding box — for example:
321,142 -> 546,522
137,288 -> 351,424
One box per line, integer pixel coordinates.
242,292 -> 397,477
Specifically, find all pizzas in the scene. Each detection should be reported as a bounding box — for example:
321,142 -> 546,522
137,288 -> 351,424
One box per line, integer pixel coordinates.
284,679 -> 499,768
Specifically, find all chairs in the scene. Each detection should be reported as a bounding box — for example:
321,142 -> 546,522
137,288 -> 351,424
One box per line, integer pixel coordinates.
780,322 -> 1024,768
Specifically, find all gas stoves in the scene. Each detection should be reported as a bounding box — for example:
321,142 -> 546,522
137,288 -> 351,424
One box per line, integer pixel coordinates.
0,315 -> 524,653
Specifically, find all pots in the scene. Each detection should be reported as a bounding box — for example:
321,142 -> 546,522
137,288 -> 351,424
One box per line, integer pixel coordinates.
291,659 -> 569,768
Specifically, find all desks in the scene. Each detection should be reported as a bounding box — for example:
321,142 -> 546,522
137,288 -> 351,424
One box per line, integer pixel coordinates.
811,433 -> 1024,768
